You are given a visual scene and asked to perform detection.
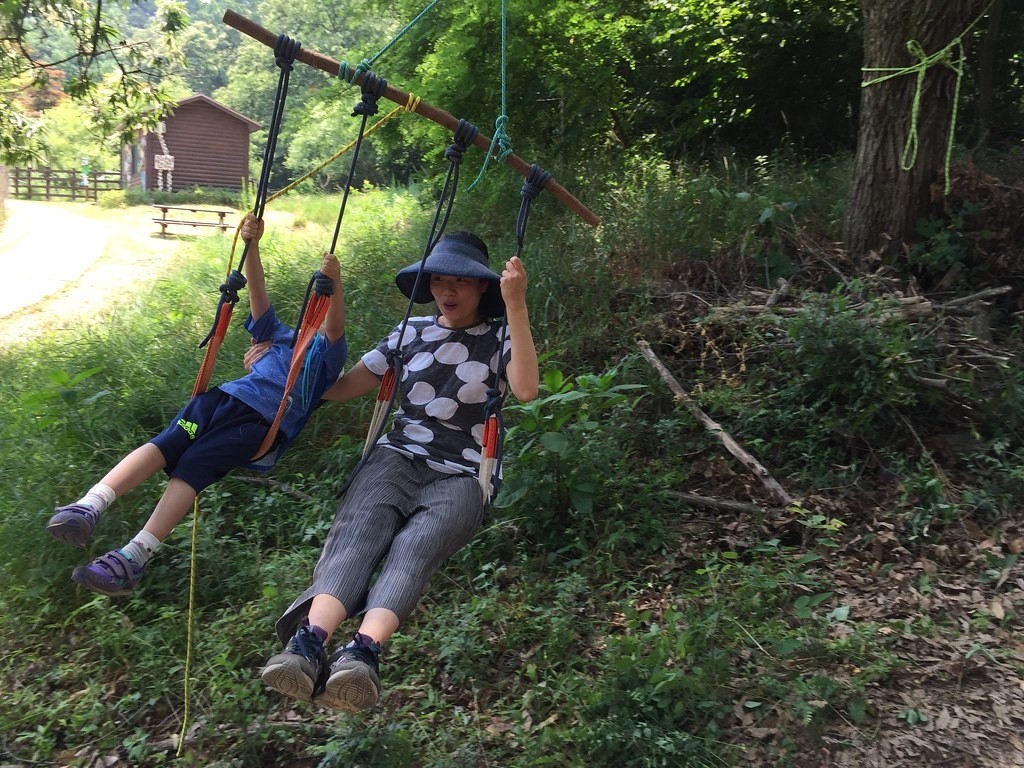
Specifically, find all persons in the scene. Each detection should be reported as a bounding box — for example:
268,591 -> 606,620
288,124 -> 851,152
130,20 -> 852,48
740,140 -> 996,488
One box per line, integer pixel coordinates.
243,232 -> 542,714
46,219 -> 349,596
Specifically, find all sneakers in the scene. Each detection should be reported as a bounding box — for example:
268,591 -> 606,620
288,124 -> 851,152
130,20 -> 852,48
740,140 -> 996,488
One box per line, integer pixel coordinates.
262,617 -> 382,716
71,548 -> 145,597
45,502 -> 100,545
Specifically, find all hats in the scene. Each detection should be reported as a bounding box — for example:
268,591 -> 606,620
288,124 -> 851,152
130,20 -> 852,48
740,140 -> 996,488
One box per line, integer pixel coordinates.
395,240 -> 506,319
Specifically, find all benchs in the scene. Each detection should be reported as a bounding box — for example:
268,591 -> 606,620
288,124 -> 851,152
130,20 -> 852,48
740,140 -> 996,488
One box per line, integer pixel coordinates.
152,218 -> 235,239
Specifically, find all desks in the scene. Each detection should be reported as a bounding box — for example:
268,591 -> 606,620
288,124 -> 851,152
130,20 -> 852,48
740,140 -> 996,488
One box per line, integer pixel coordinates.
151,204 -> 236,235
